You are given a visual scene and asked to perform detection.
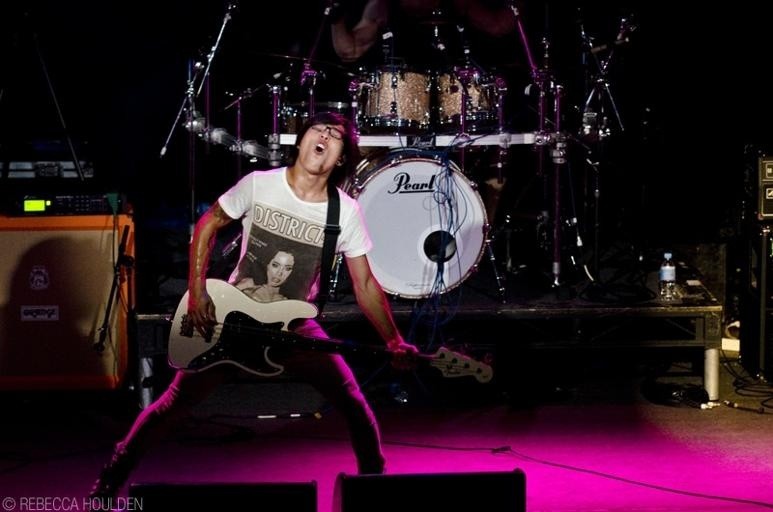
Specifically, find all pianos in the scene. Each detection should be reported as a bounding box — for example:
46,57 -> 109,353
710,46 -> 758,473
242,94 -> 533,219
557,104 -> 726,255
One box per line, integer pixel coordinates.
0,195 -> 125,214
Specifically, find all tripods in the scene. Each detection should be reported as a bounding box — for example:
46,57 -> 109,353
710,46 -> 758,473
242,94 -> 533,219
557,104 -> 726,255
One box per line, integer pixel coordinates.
452,143 -> 601,304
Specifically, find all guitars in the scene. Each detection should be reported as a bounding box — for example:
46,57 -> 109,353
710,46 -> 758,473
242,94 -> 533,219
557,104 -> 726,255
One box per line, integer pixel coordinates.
168,279 -> 493,386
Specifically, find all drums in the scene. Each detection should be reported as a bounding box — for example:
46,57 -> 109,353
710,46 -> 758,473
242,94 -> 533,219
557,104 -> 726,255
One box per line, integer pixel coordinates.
357,65 -> 434,135
435,70 -> 499,133
341,147 -> 488,300
279,101 -> 352,134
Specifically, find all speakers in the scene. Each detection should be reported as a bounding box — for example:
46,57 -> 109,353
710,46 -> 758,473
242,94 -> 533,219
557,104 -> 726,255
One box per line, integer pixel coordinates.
128,480 -> 318,512
738,217 -> 773,386
332,467 -> 526,512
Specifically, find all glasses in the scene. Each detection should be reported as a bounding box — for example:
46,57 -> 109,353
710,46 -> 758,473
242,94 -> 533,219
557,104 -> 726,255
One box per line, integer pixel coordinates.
312,122 -> 343,139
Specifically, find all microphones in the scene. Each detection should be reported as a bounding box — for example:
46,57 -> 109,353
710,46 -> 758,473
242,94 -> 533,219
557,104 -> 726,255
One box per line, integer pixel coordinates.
93,328 -> 107,353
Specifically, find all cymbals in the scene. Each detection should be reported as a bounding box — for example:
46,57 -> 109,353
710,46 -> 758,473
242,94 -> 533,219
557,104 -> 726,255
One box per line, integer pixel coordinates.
272,52 -> 345,71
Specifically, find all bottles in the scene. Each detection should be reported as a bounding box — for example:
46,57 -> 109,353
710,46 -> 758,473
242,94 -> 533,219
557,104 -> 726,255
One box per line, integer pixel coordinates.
658,252 -> 676,300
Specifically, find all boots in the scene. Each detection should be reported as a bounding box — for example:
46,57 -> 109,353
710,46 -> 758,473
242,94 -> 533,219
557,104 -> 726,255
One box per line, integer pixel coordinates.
86,444 -> 136,511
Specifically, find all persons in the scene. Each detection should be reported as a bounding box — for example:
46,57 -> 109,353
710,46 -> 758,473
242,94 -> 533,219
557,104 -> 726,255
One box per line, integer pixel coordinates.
85,111 -> 419,511
299,0 -> 400,101
